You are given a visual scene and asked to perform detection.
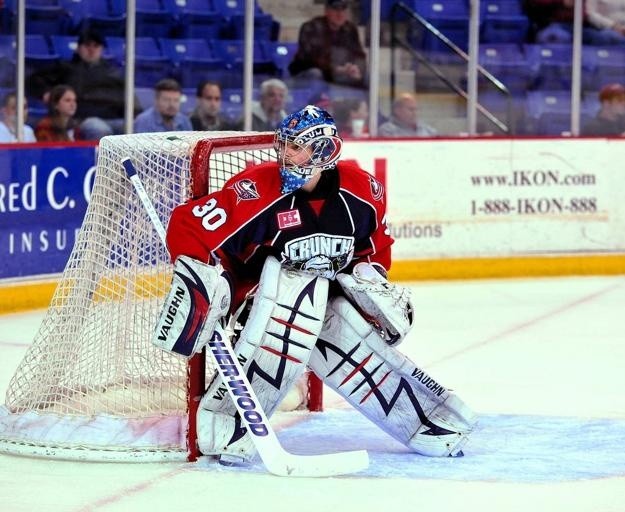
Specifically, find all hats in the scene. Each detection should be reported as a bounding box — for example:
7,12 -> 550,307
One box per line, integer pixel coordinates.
78,30 -> 108,47
598,82 -> 625,101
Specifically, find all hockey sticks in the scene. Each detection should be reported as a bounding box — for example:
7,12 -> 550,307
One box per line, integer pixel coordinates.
120,155 -> 370,476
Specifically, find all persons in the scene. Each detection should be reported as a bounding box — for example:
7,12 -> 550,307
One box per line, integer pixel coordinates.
308,92 -> 368,136
132,80 -> 192,134
35,87 -> 85,142
581,83 -> 625,135
520,0 -> 575,43
189,80 -> 238,130
0,94 -> 37,142
379,94 -> 438,135
165,105 -> 478,458
287,0 -> 366,87
55,30 -> 141,118
585,0 -> 625,35
236,80 -> 289,131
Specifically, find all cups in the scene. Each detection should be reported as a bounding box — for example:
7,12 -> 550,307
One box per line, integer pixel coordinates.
351,119 -> 364,137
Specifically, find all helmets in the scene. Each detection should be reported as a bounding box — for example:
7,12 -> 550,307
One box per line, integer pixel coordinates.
272,104 -> 343,176
